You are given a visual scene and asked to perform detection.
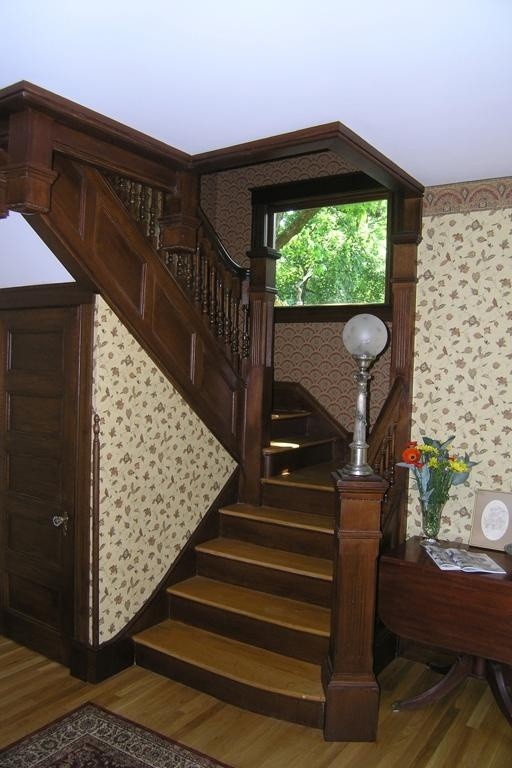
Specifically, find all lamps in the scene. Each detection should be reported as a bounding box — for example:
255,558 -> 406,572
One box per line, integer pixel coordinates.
340,314 -> 389,475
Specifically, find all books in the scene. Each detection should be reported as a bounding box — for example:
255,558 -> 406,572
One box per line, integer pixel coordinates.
445,548 -> 506,574
425,545 -> 461,571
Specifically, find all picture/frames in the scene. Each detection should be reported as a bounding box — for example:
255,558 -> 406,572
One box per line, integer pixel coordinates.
468,489 -> 512,551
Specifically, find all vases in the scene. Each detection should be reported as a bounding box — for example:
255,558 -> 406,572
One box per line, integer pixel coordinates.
417,497 -> 447,549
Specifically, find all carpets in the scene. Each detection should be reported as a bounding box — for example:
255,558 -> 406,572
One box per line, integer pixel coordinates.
0,700 -> 234,768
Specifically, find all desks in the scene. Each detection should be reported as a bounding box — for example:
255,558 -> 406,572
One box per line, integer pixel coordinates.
377,536 -> 512,727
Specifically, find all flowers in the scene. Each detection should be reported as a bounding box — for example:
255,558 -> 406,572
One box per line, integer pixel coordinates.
394,434 -> 482,532
